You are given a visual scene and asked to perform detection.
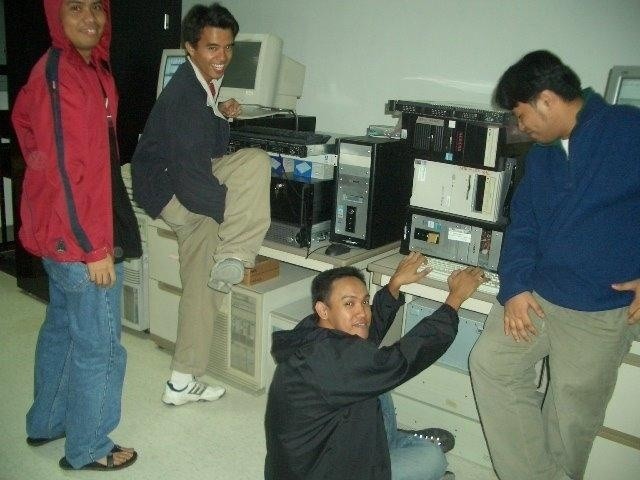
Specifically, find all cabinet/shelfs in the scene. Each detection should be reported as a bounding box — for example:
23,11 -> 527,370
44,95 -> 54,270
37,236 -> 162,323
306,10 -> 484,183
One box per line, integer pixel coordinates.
140,205 -> 405,411
368,250 -> 640,480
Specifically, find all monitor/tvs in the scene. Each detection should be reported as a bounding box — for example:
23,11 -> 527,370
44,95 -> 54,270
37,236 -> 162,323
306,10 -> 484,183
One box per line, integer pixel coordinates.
157,48 -> 188,98
603,63 -> 640,107
214,31 -> 306,121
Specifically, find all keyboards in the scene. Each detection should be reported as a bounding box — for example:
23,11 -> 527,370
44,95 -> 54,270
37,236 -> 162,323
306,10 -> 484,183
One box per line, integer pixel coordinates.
417,254 -> 500,296
230,122 -> 331,146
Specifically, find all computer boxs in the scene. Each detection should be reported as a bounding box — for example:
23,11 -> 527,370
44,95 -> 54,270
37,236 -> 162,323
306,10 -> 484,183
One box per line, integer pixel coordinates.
329,134 -> 405,250
406,153 -> 518,226
398,111 -> 506,172
399,205 -> 505,274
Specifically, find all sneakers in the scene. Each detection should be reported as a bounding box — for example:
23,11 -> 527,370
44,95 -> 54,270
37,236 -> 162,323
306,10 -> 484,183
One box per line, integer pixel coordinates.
206,259 -> 245,293
399,428 -> 455,454
161,380 -> 225,406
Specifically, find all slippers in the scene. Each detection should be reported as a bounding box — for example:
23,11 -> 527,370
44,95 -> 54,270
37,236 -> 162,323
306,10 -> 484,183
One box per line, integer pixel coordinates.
58,445 -> 137,470
27,437 -> 61,446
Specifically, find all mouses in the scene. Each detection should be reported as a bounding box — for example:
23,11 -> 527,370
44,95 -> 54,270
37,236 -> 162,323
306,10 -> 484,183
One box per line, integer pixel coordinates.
326,243 -> 349,256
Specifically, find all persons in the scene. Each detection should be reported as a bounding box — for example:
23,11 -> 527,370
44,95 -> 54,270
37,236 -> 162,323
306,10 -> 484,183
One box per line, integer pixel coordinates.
130,3 -> 271,404
11,2 -> 139,471
467,49 -> 638,480
263,252 -> 492,479
